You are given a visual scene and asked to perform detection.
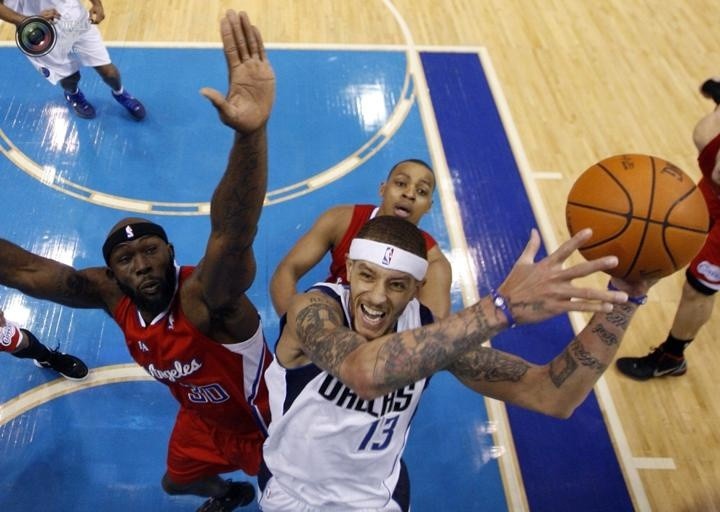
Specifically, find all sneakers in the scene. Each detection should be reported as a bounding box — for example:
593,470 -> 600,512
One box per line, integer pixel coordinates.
616,343 -> 688,382
33,347 -> 90,382
63,87 -> 97,121
195,480 -> 257,512
111,87 -> 147,122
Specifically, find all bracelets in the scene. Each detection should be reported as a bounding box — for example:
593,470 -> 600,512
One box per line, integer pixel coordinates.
605,281 -> 648,307
492,288 -> 518,331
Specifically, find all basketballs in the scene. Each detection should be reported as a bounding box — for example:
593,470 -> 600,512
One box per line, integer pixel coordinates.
565,153 -> 711,284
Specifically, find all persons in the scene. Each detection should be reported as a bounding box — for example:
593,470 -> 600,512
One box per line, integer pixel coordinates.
0,306 -> 90,381
0,1 -> 147,122
617,77 -> 719,382
270,159 -> 453,320
0,10 -> 278,511
260,214 -> 660,510
